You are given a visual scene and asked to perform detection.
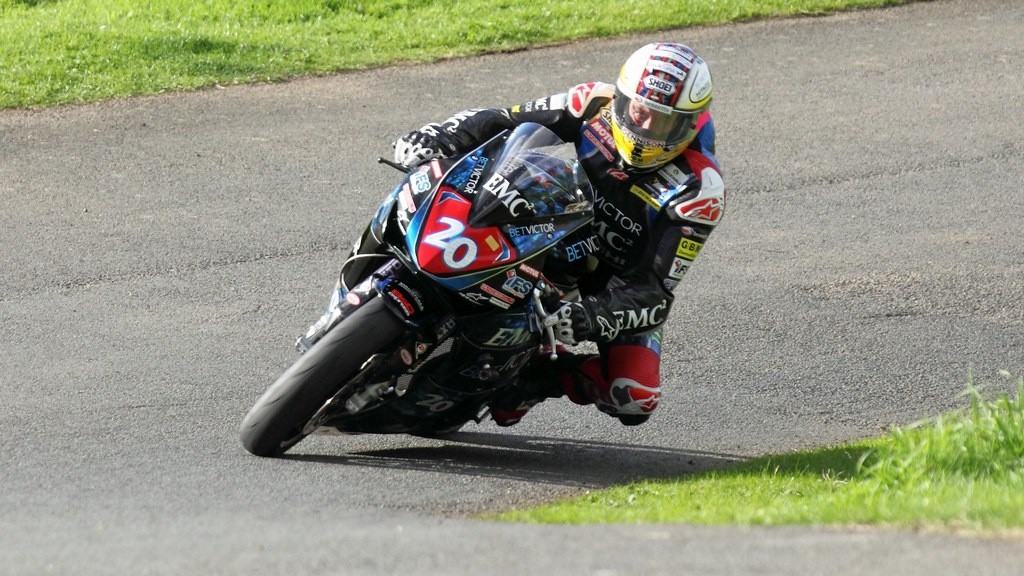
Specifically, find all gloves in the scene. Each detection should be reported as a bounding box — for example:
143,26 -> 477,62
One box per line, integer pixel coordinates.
391,130 -> 447,171
552,297 -> 609,348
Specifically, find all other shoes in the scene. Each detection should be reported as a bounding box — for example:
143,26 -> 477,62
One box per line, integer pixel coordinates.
489,362 -> 555,426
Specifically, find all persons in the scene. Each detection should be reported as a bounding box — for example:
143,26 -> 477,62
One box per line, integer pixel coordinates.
391,41 -> 728,428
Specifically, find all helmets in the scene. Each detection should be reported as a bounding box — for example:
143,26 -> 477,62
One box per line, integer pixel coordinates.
610,41 -> 715,175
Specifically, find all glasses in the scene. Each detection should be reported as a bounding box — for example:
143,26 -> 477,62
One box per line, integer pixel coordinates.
630,102 -> 672,128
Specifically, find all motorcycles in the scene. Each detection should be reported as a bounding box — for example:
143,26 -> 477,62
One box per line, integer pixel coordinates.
235,118 -> 607,458
